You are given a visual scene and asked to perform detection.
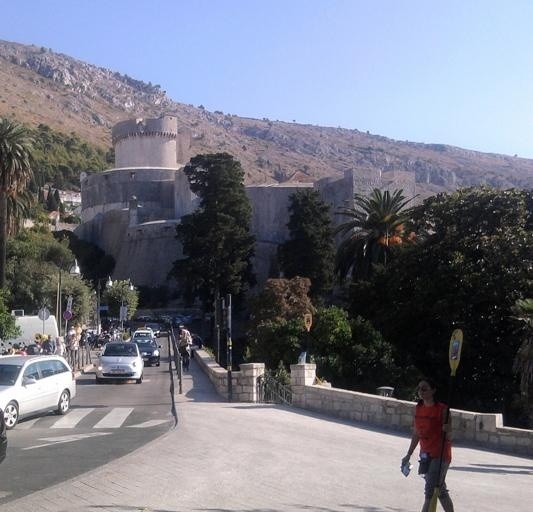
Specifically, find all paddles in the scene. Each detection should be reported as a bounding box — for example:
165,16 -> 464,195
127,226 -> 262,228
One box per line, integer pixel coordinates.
428,328 -> 464,512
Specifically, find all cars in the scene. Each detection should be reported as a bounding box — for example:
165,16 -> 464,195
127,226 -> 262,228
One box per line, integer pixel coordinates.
92,313 -> 192,384
0,409 -> 8,461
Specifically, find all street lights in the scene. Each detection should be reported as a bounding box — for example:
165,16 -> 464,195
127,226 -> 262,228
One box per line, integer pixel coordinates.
120,282 -> 137,331
57,257 -> 81,337
96,273 -> 113,337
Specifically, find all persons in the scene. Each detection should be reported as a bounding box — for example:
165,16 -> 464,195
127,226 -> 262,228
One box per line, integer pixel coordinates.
177,323 -> 191,372
0,323 -> 123,368
401,378 -> 454,511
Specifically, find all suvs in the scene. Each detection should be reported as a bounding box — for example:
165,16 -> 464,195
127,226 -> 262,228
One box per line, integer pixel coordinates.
0,356 -> 75,428
